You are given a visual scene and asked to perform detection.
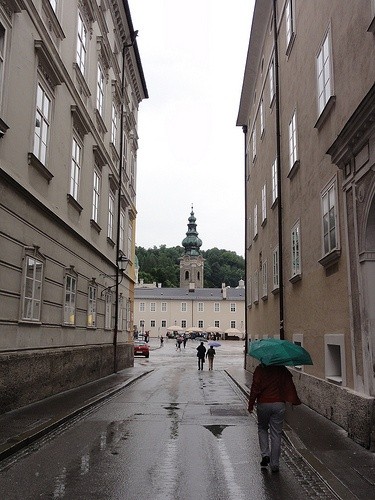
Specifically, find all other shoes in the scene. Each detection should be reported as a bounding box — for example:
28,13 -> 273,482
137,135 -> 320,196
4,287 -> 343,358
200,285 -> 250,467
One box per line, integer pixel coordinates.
271,468 -> 279,474
260,456 -> 271,466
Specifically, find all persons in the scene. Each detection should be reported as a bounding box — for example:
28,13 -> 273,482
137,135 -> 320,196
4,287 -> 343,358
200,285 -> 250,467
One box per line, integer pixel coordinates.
134,330 -> 138,339
176,335 -> 181,351
248,364 -> 301,472
214,333 -> 219,341
197,342 -> 206,370
206,346 -> 216,371
144,332 -> 148,342
183,333 -> 187,348
161,337 -> 163,343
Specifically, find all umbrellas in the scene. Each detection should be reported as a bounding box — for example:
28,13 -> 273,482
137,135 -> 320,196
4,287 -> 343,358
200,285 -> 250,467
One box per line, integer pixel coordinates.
208,341 -> 221,347
248,339 -> 313,367
167,326 -> 241,334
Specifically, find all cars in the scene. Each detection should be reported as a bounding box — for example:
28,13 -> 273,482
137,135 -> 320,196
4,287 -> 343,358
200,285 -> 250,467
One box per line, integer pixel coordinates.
133,339 -> 149,358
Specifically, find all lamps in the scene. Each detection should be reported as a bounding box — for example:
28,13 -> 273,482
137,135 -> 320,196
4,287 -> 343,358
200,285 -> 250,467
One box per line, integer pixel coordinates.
101,254 -> 130,296
